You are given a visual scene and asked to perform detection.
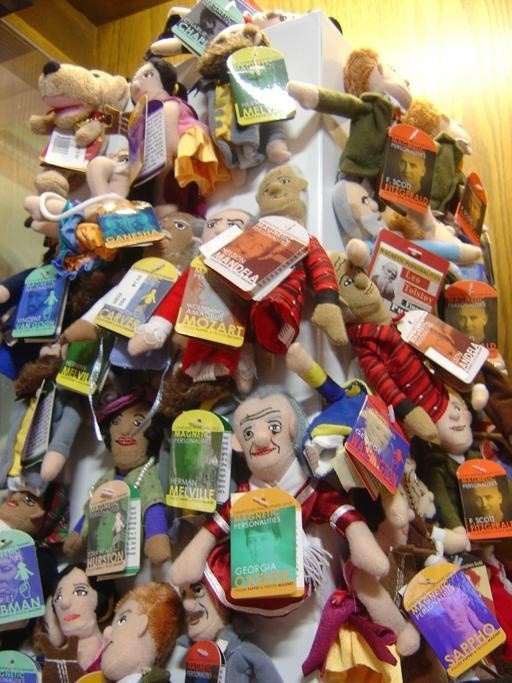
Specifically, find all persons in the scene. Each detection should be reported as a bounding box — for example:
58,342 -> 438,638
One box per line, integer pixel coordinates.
0,7 -> 512,683
